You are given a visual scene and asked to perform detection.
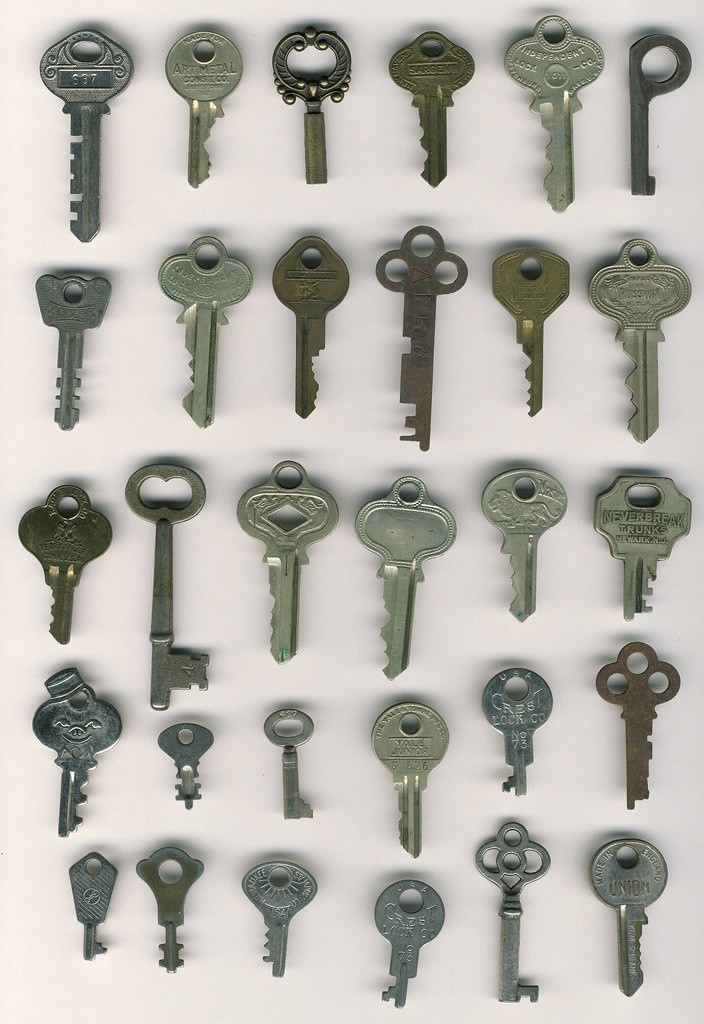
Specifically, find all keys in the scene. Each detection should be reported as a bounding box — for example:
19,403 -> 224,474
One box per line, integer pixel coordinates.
13,15 -> 691,1012
